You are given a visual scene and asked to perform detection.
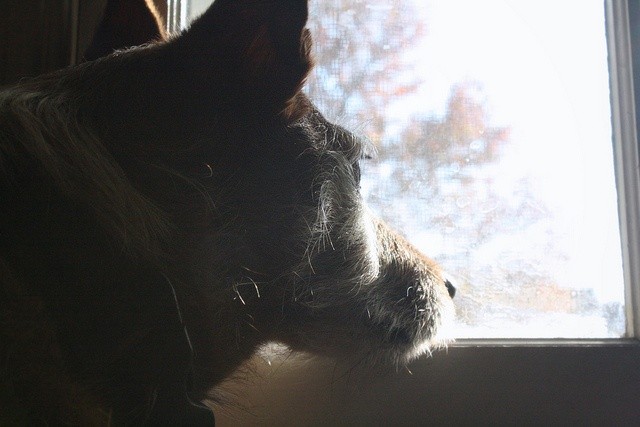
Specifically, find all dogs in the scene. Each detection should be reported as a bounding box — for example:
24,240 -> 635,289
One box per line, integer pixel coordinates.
0,2 -> 457,425
